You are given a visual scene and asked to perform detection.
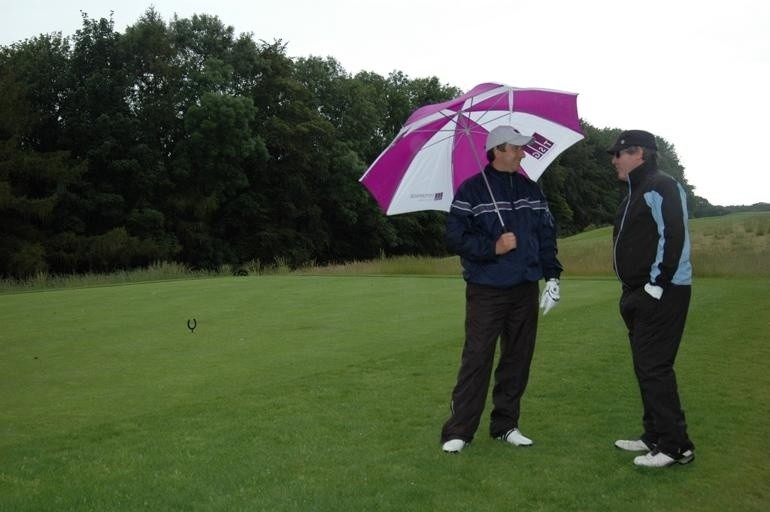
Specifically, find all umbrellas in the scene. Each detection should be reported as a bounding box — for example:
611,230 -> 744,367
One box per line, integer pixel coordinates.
357,82 -> 589,234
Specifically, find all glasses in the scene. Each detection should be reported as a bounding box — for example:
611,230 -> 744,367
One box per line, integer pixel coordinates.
616,148 -> 640,159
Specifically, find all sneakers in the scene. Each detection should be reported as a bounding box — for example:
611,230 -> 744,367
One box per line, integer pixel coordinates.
614,437 -> 658,451
442,438 -> 466,453
491,426 -> 534,447
633,447 -> 695,468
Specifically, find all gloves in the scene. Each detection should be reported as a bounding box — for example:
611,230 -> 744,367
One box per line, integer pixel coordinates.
640,280 -> 667,305
538,278 -> 561,316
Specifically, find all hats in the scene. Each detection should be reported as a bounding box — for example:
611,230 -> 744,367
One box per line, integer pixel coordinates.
606,129 -> 658,155
485,125 -> 533,153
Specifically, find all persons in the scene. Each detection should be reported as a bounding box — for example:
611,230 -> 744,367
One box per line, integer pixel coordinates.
606,130 -> 695,470
439,125 -> 564,453
